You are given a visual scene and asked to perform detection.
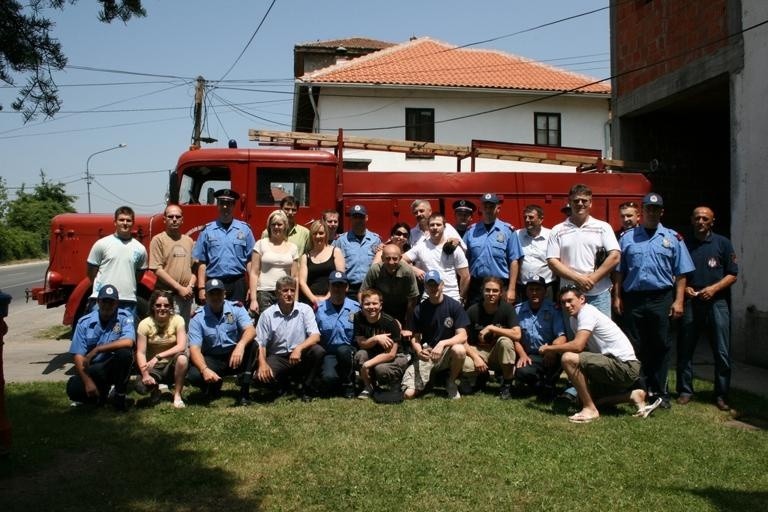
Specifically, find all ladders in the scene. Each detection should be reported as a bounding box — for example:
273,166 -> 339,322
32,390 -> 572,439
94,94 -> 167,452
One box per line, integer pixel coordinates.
247,127 -> 624,166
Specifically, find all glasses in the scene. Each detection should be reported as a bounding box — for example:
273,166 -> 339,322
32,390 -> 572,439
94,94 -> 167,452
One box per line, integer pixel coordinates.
561,284 -> 578,290
155,303 -> 170,308
395,230 -> 410,239
620,202 -> 636,208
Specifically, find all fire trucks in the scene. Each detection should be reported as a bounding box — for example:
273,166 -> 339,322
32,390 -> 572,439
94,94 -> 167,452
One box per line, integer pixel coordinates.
26,127 -> 653,327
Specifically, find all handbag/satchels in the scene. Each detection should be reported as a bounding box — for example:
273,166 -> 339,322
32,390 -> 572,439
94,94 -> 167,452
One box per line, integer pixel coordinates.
596,247 -> 608,269
373,386 -> 406,403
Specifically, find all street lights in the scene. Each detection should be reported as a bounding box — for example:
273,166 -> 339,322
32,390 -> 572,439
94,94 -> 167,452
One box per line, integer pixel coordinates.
86,143 -> 127,213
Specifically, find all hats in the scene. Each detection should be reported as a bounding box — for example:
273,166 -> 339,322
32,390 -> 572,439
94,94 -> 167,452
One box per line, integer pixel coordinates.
205,279 -> 224,292
423,270 -> 440,283
100,285 -> 118,300
213,189 -> 240,203
643,193 -> 664,205
351,205 -> 367,216
329,271 -> 348,285
453,200 -> 476,212
561,203 -> 571,212
481,193 -> 499,203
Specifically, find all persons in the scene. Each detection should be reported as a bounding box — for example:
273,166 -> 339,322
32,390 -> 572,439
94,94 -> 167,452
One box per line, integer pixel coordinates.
66,190 -> 256,411
545,183 -> 622,403
539,284 -> 661,423
246,192 -> 552,400
615,192 -> 739,411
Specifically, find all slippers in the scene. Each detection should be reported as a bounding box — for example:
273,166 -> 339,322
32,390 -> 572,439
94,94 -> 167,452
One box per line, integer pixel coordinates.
632,397 -> 662,420
569,410 -> 600,424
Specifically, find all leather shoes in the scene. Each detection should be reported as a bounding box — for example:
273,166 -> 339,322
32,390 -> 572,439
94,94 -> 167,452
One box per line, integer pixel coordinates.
659,396 -> 671,409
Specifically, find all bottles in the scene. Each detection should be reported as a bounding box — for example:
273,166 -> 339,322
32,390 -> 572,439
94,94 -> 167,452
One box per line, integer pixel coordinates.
422,342 -> 433,364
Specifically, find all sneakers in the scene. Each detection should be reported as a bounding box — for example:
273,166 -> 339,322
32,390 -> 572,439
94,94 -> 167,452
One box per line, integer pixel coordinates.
151,390 -> 161,401
500,385 -> 513,399
422,385 -> 434,399
357,389 -> 370,399
344,384 -> 355,397
174,398 -> 185,408
677,395 -> 691,404
302,383 -> 312,401
451,390 -> 461,400
713,392 -> 730,410
544,382 -> 555,395
239,388 -> 249,405
